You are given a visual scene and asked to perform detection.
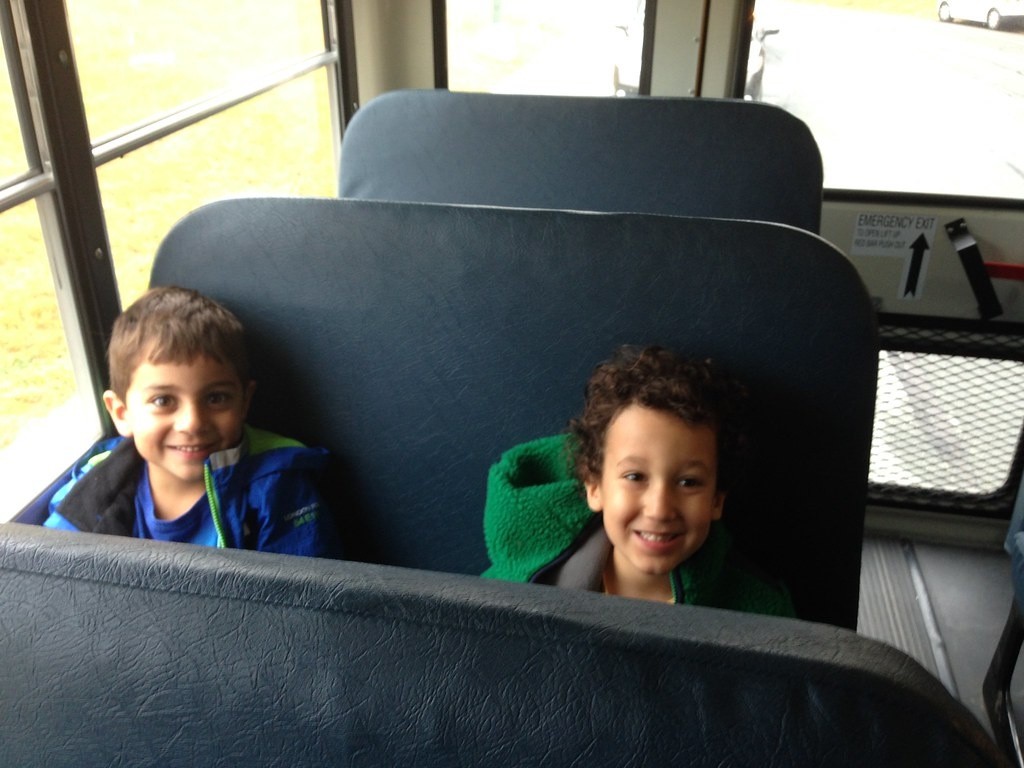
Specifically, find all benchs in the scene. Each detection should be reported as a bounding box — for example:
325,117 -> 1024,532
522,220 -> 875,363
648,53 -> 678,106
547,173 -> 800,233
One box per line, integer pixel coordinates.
336,87 -> 824,236
0,523 -> 1004,768
148,197 -> 878,635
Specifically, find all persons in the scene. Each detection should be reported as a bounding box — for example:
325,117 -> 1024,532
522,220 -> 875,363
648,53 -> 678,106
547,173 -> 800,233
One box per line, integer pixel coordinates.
41,284 -> 333,556
478,342 -> 797,619
